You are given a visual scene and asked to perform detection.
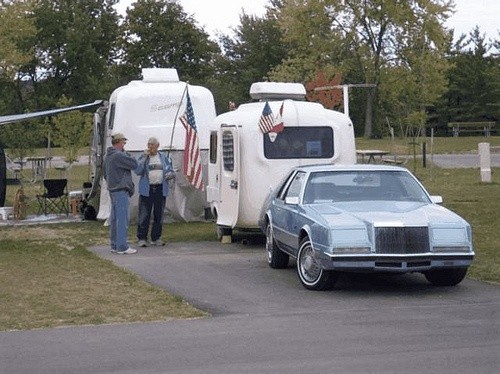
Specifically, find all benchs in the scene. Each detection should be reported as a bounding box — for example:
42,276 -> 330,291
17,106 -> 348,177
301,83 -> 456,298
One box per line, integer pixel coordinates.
381,158 -> 404,167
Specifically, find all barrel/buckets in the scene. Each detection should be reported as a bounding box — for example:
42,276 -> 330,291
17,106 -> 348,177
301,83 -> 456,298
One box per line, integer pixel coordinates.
0,207 -> 14,221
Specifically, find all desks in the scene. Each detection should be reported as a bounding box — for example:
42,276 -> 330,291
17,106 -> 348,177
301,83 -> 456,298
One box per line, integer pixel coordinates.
357,149 -> 390,163
28,156 -> 52,185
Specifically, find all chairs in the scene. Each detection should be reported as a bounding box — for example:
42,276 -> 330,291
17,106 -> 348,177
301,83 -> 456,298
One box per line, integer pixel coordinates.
311,183 -> 338,200
36,177 -> 69,215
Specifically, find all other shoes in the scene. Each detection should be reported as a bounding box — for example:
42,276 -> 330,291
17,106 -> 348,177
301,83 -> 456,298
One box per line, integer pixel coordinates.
111,247 -> 136,254
150,239 -> 168,246
137,239 -> 148,247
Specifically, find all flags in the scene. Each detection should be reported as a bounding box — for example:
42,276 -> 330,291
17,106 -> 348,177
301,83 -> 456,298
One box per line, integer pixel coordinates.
258,101 -> 275,133
269,103 -> 284,132
180,90 -> 205,192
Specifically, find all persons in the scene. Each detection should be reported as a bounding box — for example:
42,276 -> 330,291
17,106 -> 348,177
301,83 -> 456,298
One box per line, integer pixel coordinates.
134,136 -> 177,247
103,133 -> 139,255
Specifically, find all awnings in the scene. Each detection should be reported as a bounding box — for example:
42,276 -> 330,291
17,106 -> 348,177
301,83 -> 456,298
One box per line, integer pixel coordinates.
0,99 -> 104,126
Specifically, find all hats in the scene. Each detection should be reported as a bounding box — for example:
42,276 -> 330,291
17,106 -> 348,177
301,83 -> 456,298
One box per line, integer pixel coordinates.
111,132 -> 128,141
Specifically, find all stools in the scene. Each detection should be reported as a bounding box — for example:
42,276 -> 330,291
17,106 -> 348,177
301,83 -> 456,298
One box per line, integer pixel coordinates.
1,207 -> 14,221
69,191 -> 83,215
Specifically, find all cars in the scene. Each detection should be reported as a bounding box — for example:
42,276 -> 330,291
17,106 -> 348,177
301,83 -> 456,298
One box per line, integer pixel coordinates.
258,164 -> 474,292
